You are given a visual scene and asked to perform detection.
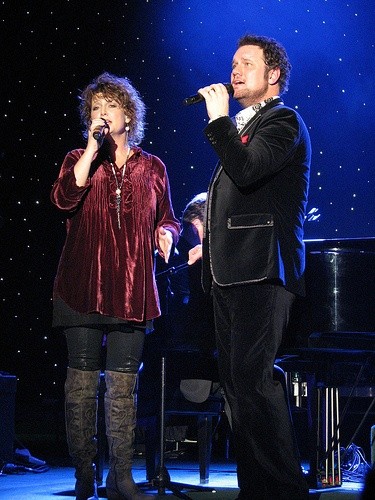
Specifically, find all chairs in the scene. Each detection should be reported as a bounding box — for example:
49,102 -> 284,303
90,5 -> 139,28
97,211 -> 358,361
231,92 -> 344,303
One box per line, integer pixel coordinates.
155,271 -> 308,478
293,244 -> 375,485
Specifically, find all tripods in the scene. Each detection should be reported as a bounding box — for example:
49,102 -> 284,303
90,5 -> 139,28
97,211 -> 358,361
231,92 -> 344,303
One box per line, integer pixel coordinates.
135,262 -> 218,500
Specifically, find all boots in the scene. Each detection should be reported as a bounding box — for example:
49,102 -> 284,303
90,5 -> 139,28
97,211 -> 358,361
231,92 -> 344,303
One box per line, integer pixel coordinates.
66,365 -> 102,499
103,370 -> 154,499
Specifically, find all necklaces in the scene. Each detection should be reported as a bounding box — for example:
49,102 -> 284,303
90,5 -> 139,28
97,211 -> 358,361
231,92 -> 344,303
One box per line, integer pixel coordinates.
108,148 -> 131,229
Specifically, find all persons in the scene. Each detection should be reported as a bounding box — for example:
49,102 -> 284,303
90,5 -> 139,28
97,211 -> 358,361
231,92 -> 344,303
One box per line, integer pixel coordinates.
153,191 -> 233,434
187,34 -> 309,500
49,73 -> 183,499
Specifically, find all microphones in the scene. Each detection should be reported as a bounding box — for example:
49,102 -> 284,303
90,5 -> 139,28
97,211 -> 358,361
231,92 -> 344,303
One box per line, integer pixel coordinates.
182,83 -> 233,106
93,118 -> 107,140
